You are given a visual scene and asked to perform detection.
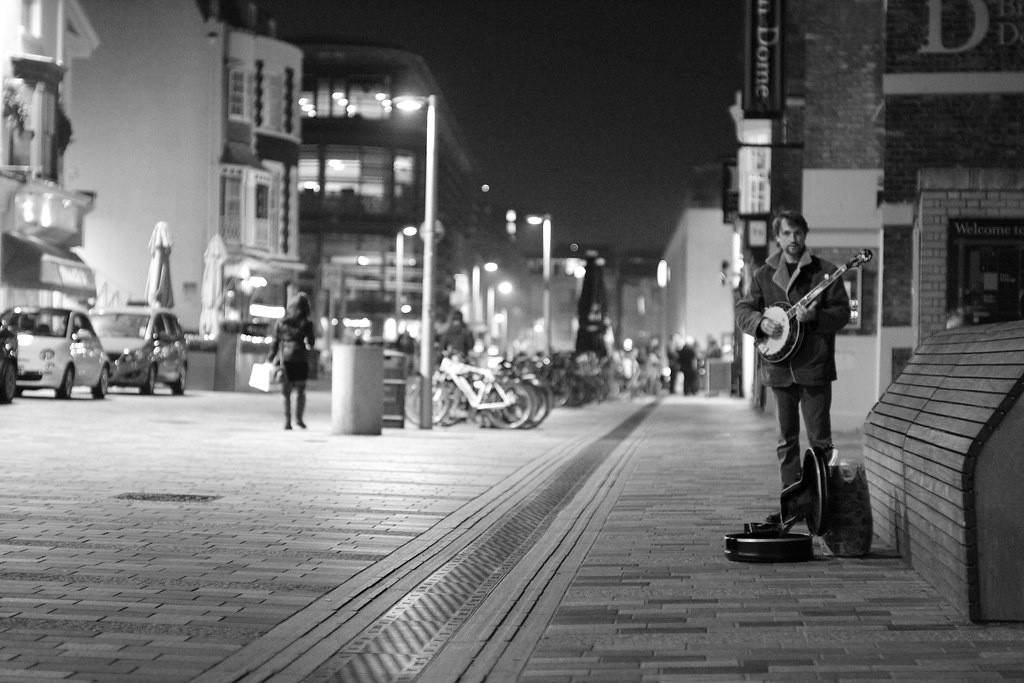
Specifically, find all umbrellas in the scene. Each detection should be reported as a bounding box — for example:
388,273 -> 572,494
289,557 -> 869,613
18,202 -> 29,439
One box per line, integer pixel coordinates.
198,228 -> 232,341
144,219 -> 176,307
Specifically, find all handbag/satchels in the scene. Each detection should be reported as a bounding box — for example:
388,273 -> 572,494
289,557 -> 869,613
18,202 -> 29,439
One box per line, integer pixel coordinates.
281,357 -> 309,380
250,362 -> 283,391
823,463 -> 873,559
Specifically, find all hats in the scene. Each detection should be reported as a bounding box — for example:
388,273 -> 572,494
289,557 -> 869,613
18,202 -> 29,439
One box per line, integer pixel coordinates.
452,310 -> 462,320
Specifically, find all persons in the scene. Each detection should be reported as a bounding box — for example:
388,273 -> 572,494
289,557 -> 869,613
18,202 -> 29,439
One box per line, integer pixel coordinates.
617,331 -> 724,398
737,214 -> 851,524
269,292 -> 314,430
442,311 -> 473,366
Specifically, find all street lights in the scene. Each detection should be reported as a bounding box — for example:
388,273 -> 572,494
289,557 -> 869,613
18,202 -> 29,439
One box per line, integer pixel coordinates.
392,94 -> 435,429
395,226 -> 418,335
487,283 -> 513,327
473,263 -> 498,322
527,212 -> 552,364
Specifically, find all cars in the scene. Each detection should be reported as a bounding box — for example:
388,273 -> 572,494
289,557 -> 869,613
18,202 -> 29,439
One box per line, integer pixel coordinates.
0,305 -> 109,403
89,306 -> 188,395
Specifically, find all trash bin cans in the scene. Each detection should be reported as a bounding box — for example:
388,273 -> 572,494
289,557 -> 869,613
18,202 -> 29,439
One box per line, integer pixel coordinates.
330,345 -> 383,435
381,349 -> 408,429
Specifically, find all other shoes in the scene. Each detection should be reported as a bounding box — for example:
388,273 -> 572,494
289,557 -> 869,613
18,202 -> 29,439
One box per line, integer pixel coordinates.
297,419 -> 306,428
285,424 -> 292,429
765,511 -> 804,523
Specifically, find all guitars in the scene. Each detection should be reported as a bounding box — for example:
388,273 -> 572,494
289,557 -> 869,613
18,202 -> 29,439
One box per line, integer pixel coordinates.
752,247 -> 875,366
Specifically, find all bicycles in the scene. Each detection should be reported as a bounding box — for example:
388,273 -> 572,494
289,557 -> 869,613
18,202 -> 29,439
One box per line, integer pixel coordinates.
404,344 -> 606,428
